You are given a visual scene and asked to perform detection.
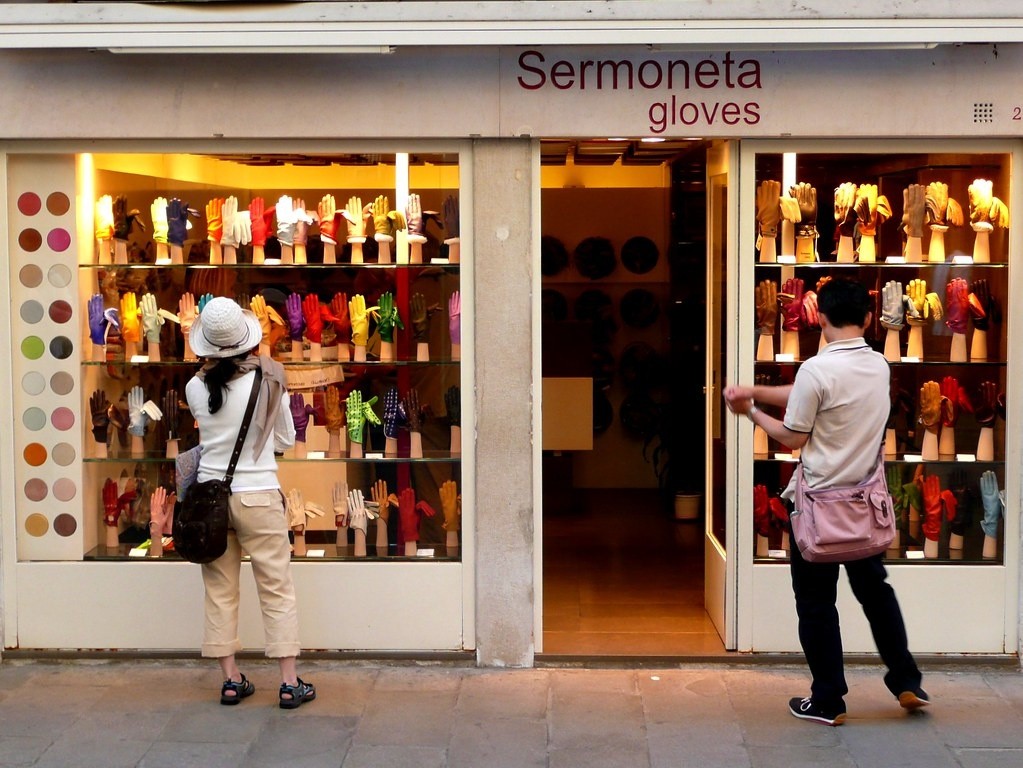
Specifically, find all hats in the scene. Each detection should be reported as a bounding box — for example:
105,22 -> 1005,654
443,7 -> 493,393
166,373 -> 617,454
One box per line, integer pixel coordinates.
543,236 -> 681,441
189,296 -> 263,358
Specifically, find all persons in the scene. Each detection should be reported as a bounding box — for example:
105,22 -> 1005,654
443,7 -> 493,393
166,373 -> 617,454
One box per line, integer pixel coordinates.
719,278 -> 931,726
184,297 -> 317,710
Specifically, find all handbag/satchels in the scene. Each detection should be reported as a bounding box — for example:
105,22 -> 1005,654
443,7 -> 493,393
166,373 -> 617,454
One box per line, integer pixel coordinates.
791,446 -> 897,563
171,474 -> 233,562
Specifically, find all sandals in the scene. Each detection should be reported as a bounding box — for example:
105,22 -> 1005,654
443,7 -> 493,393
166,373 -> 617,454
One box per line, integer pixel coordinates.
278,677 -> 315,709
219,674 -> 254,705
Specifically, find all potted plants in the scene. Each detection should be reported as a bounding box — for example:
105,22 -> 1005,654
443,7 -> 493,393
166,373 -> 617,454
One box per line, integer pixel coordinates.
640,407 -> 704,520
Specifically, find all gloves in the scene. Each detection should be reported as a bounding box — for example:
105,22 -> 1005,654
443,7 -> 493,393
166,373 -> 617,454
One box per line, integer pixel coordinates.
92,185 -> 460,553
746,171 -> 1005,560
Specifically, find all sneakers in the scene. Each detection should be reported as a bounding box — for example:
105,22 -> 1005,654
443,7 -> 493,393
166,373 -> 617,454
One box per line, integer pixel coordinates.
897,687 -> 930,709
787,696 -> 847,726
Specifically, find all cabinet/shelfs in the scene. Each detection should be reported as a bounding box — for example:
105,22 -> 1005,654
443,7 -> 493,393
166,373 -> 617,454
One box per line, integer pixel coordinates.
80,259 -> 1007,567
